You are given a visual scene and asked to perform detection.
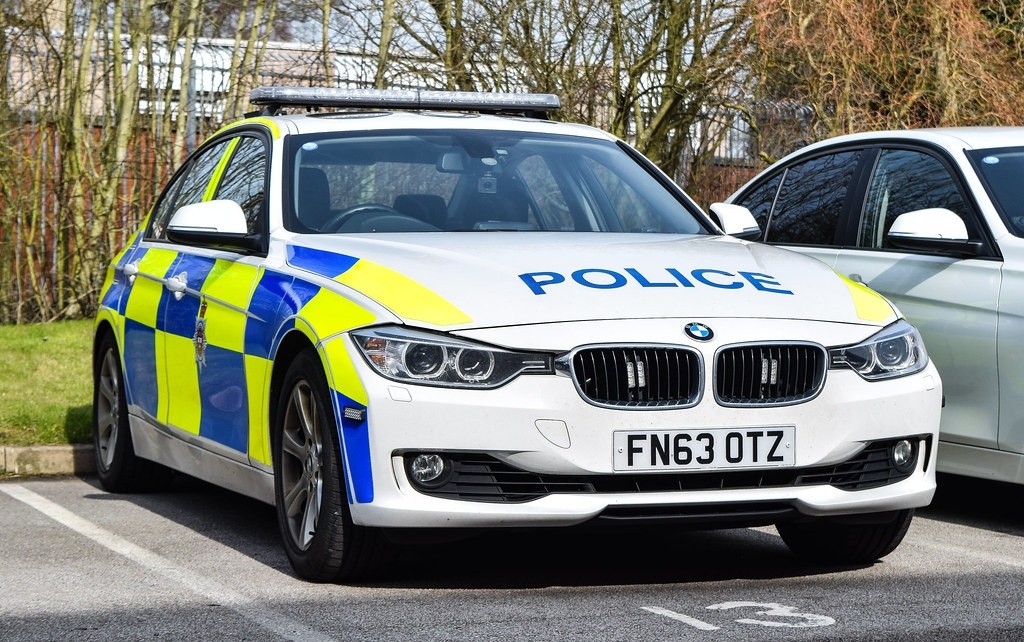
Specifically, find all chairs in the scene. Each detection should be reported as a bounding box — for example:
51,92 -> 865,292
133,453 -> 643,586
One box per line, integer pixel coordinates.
394,193 -> 448,227
299,167 -> 331,224
465,182 -> 529,231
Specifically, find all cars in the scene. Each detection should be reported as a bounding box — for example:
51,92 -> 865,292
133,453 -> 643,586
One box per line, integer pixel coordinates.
89,86 -> 948,590
702,121 -> 1024,487
